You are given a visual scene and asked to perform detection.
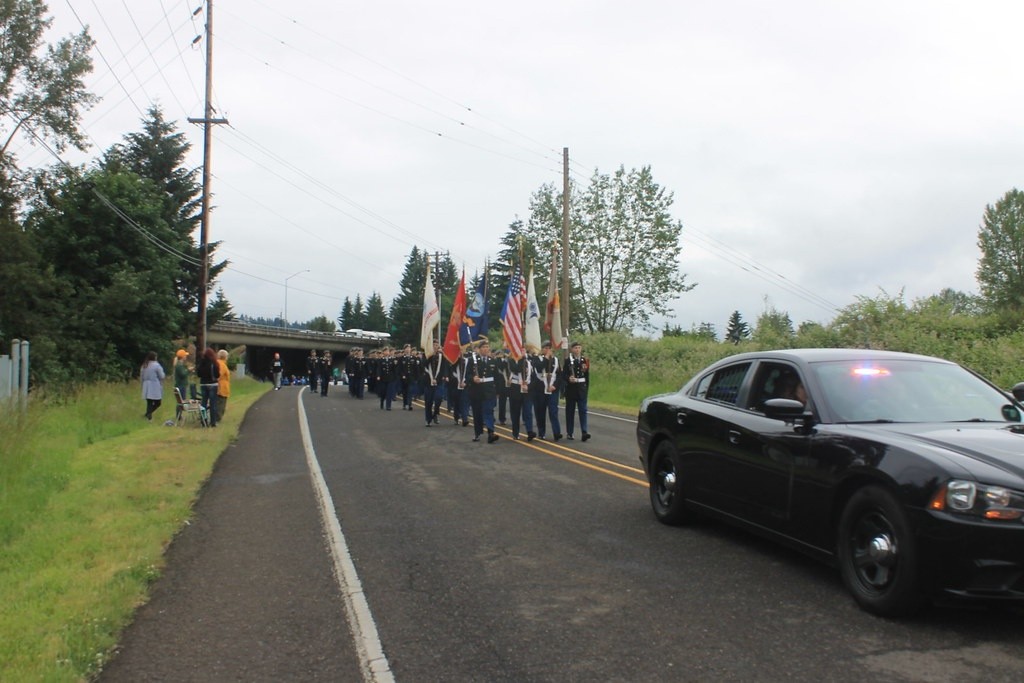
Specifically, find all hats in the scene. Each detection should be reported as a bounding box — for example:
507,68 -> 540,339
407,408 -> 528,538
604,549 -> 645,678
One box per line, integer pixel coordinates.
176,349 -> 189,358
311,340 -> 580,355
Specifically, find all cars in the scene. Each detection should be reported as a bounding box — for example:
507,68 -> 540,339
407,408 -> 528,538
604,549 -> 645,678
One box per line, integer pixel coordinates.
637,347 -> 1024,620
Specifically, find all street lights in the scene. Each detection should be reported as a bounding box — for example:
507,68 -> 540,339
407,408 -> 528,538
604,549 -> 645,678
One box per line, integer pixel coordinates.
285,269 -> 311,330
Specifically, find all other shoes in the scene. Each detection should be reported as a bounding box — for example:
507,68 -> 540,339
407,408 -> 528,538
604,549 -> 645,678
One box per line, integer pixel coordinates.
310,388 -> 592,444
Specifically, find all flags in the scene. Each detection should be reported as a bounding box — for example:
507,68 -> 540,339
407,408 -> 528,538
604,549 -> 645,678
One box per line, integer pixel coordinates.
544,252 -> 562,350
499,258 -> 527,360
421,265 -> 440,358
442,273 -> 465,364
524,269 -> 541,350
457,274 -> 488,348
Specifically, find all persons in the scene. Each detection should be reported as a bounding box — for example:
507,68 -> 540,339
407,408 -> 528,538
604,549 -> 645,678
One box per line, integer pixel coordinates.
282,375 -> 310,385
140,351 -> 166,420
563,342 -> 591,442
270,352 -> 283,391
216,350 -> 230,421
345,343 -> 543,426
792,377 -> 813,415
531,340 -> 563,441
333,368 -> 337,385
508,346 -> 536,441
173,348 -> 193,421
465,341 -> 499,444
320,350 -> 332,396
307,350 -> 320,393
197,349 -> 220,427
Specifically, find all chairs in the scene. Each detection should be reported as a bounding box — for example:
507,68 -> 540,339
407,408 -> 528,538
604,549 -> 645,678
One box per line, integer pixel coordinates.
174,387 -> 208,426
190,384 -> 211,419
